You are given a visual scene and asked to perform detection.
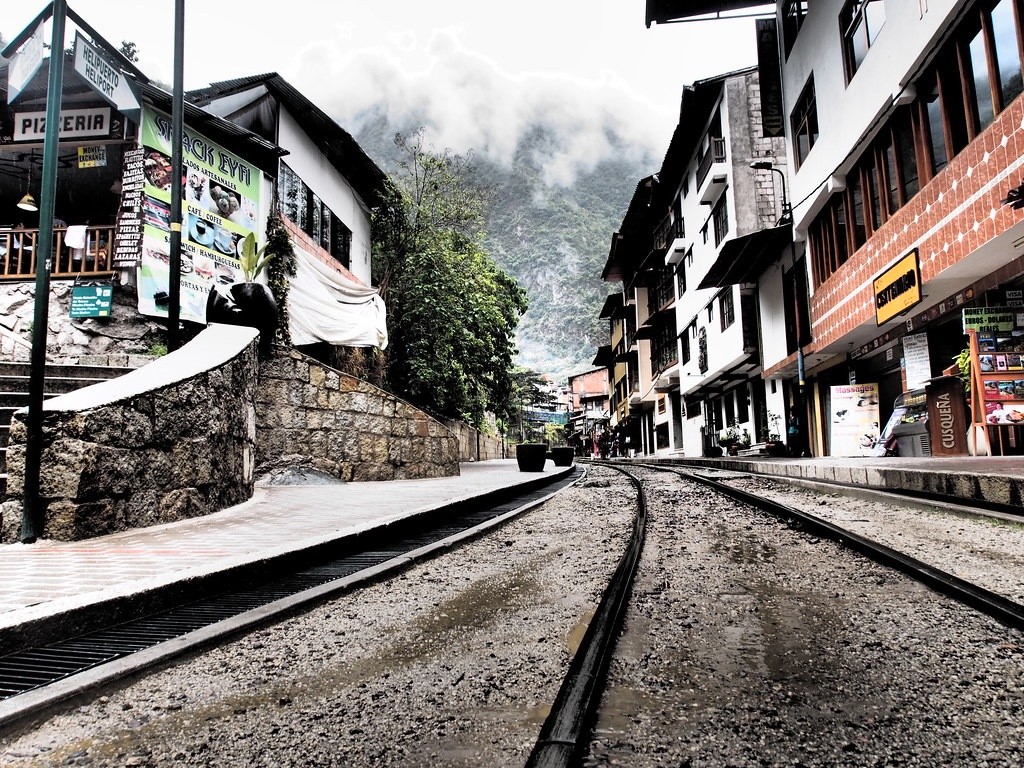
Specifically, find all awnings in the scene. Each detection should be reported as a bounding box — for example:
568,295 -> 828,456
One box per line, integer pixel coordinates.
590,246 -> 667,321
630,320 -> 663,343
589,342 -> 612,367
641,306 -> 674,329
562,417 -> 611,442
695,215 -> 798,294
645,1 -> 783,34
614,351 -> 637,365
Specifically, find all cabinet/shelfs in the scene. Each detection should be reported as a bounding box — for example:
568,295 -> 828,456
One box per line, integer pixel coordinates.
968,329 -> 1024,457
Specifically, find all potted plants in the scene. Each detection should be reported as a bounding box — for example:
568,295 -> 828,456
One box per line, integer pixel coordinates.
230,232 -> 274,310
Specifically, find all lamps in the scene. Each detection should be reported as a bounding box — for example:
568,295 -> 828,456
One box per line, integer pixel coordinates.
16,148 -> 39,212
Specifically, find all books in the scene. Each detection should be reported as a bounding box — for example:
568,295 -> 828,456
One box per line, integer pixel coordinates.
976,329 -> 1024,424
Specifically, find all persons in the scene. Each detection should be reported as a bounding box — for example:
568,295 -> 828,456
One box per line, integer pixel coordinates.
5,215 -> 115,274
992,403 -> 1004,422
